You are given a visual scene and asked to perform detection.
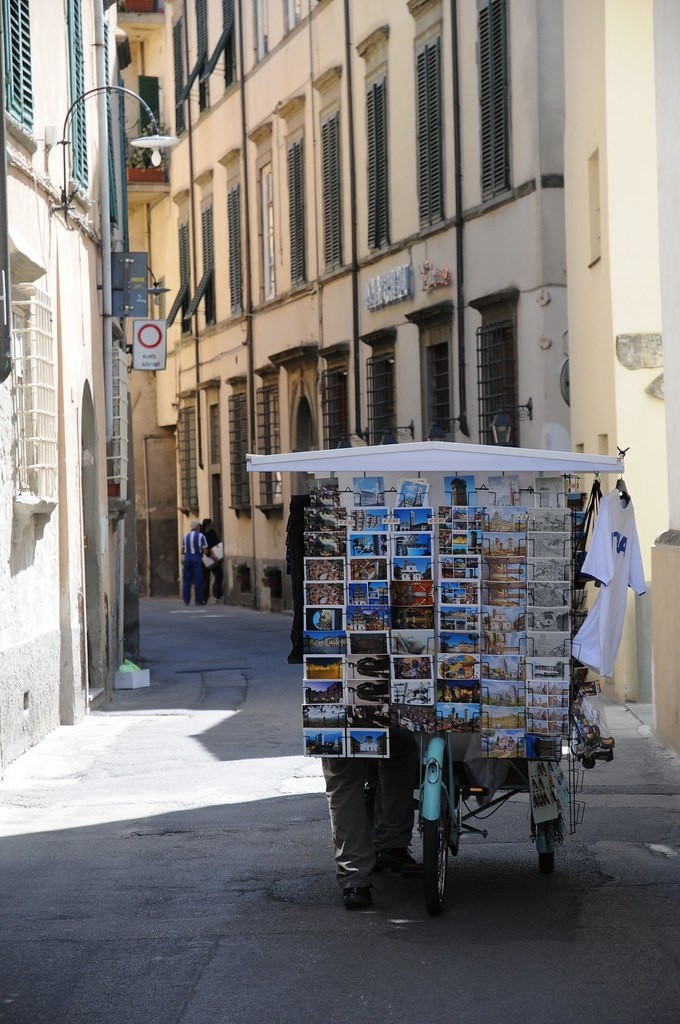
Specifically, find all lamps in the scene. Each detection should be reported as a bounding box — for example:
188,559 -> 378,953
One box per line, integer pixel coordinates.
147,267 -> 171,305
492,399 -> 534,447
49,84 -> 181,231
380,422 -> 414,444
424,414 -> 468,441
337,429 -> 369,449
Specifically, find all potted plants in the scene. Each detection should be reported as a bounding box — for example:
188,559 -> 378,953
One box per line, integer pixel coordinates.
124,121 -> 173,182
261,567 -> 278,586
236,564 -> 250,581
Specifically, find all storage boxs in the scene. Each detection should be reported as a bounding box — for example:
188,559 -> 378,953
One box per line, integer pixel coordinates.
114,669 -> 151,690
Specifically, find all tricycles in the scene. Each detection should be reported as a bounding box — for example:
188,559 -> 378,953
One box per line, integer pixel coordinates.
248,441 -> 626,919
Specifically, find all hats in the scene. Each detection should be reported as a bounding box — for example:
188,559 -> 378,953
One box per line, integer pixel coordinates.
191,520 -> 200,531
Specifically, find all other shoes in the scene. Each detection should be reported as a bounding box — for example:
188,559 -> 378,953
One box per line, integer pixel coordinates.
342,884 -> 374,911
377,846 -> 405,872
288,646 -> 302,664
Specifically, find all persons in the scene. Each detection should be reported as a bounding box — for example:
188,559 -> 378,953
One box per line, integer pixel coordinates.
181,519 -> 223,607
349,657 -> 390,679
349,682 -> 388,703
352,708 -> 372,727
321,757 -> 415,908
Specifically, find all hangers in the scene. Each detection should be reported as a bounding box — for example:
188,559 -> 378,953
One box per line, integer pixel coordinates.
612,455 -> 632,504
